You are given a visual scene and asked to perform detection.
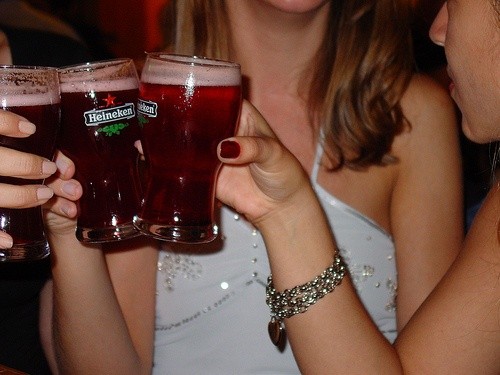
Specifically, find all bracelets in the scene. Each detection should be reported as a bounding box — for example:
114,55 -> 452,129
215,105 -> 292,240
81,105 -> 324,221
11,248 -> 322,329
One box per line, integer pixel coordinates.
262,254 -> 348,347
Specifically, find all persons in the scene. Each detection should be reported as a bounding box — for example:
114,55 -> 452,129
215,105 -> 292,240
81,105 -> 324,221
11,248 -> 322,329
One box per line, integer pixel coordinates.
0,15 -> 103,375
137,1 -> 499,374
43,0 -> 466,375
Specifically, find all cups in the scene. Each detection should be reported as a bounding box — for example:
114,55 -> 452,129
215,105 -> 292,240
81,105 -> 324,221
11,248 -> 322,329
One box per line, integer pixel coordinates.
0,63 -> 62,262
53,54 -> 148,246
131,51 -> 241,246
